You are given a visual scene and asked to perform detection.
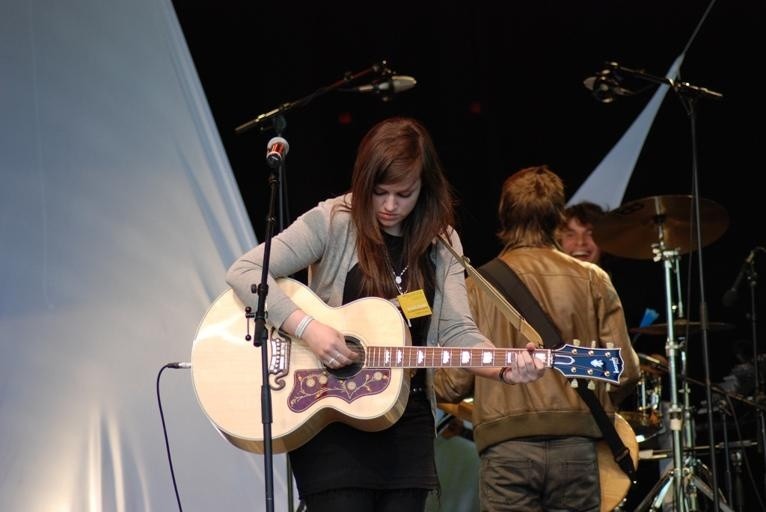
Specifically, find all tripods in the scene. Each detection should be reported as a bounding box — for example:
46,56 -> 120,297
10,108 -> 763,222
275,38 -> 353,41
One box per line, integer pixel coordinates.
635,263 -> 728,512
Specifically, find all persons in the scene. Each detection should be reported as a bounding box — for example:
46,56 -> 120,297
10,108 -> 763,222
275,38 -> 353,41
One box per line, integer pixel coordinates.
552,202 -> 670,378
225,119 -> 544,512
435,166 -> 640,512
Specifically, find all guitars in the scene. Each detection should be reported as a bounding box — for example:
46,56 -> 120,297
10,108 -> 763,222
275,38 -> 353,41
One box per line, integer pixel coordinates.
192,278 -> 625,455
439,392 -> 639,507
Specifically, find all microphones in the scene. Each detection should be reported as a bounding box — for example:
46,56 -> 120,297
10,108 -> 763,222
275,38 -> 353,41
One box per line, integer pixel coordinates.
267,136 -> 289,170
350,75 -> 416,94
583,76 -> 636,97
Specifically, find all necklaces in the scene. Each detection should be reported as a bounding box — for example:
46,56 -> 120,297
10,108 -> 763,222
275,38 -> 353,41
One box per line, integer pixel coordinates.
385,255 -> 414,329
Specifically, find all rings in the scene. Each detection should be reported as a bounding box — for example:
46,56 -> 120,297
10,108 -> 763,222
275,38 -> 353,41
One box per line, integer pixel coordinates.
328,358 -> 334,364
334,354 -> 341,360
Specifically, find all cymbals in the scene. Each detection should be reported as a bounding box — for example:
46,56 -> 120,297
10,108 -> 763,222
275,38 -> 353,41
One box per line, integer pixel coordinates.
590,194 -> 728,259
630,319 -> 728,334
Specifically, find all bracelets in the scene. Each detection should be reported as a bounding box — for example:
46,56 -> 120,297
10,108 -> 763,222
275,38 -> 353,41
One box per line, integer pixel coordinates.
295,314 -> 314,340
499,367 -> 505,384
